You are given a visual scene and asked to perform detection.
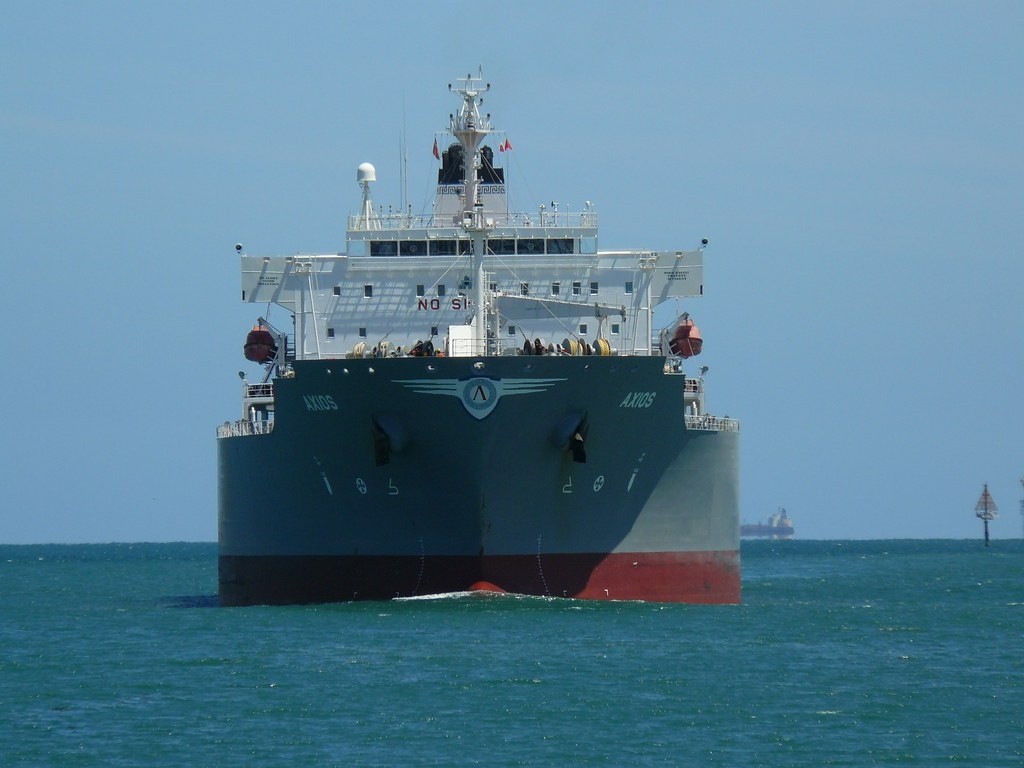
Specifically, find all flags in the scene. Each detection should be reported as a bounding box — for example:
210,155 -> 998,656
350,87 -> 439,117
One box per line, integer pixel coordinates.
504,138 -> 512,151
499,143 -> 505,152
432,137 -> 440,160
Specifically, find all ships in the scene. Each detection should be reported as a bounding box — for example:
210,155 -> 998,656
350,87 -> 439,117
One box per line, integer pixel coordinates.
214,63 -> 747,603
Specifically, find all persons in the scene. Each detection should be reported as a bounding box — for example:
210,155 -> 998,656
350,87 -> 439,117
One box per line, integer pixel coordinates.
663,356 -> 740,433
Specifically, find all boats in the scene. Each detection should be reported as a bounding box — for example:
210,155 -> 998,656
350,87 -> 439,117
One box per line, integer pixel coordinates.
666,318 -> 703,359
241,325 -> 278,365
